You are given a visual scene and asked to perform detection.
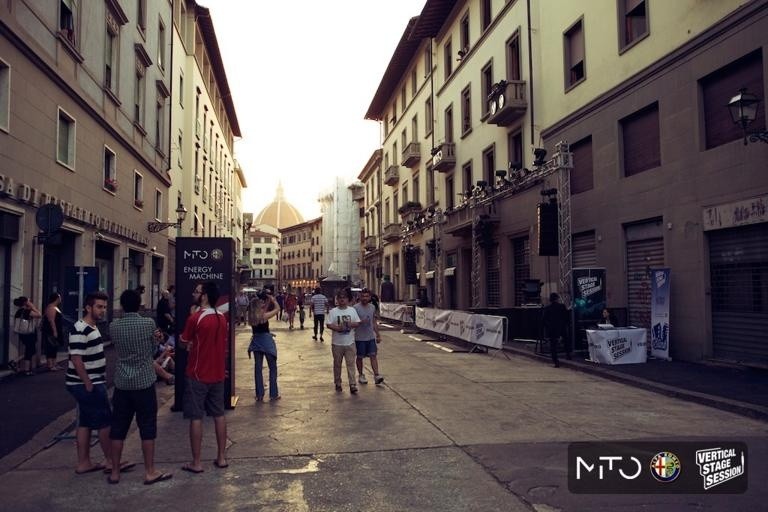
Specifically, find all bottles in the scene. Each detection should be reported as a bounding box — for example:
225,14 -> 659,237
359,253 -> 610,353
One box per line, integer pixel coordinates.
366,315 -> 371,327
337,315 -> 351,332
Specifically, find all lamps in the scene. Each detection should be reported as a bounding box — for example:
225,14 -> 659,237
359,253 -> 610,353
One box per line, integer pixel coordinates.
477,180 -> 486,191
509,161 -> 520,177
532,148 -> 547,166
407,208 -> 435,225
496,170 -> 506,179
148,204 -> 188,233
728,86 -> 767,145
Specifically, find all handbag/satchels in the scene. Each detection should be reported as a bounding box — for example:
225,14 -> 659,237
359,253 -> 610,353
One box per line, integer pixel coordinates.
13,317 -> 34,333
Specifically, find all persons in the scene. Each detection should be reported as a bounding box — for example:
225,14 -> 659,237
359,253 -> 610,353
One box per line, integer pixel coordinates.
8,296 -> 41,377
598,307 -> 618,327
43,292 -> 64,370
276,288 -> 328,342
326,289 -> 363,393
248,290 -> 281,401
179,282 -> 228,473
354,288 -> 384,384
138,284 -> 176,387
235,289 -> 263,327
351,291 -> 383,345
109,287 -> 172,485
66,292 -> 136,474
541,292 -> 572,368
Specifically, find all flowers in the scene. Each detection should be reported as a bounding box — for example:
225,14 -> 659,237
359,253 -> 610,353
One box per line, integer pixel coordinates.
106,177 -> 117,191
135,200 -> 142,207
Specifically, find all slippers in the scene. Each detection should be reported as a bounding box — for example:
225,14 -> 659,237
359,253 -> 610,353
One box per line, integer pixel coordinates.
77,461 -> 134,483
145,470 -> 172,485
181,463 -> 203,472
215,459 -> 228,468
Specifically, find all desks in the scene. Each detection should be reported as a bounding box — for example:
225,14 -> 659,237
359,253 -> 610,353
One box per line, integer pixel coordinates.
585,326 -> 648,365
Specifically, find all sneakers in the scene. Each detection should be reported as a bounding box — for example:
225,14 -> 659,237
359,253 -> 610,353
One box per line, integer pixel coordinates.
359,375 -> 368,383
256,395 -> 282,402
9,359 -> 64,375
555,361 -> 560,367
313,335 -> 324,342
335,383 -> 358,393
289,325 -> 303,329
374,375 -> 384,384
167,375 -> 174,386
